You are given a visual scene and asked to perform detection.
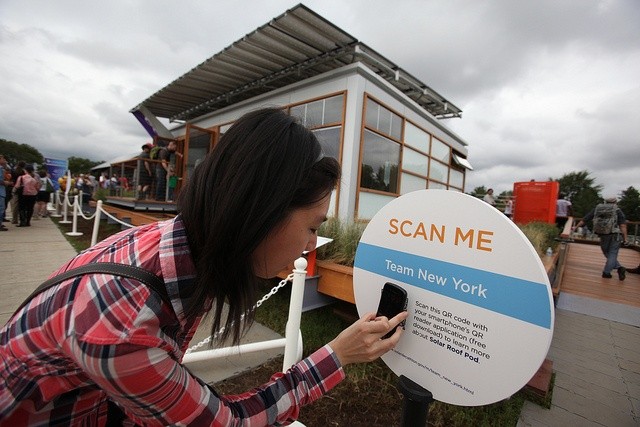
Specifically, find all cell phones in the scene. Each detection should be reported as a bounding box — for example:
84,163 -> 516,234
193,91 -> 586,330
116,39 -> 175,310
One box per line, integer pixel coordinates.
376,282 -> 407,340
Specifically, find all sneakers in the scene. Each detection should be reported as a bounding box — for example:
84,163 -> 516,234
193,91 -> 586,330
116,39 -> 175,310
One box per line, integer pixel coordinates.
617,267 -> 625,281
603,272 -> 612,278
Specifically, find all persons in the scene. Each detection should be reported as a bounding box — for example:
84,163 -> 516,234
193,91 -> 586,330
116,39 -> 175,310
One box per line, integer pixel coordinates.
504,196 -> 513,218
553,194 -> 573,233
482,188 -> 496,208
1,105 -> 409,427
154,141 -> 184,201
39,163 -> 50,215
574,197 -> 629,281
67,169 -> 132,195
1,154 -> 9,231
14,165 -> 38,227
9,161 -> 26,224
36,171 -> 55,218
137,145 -> 153,200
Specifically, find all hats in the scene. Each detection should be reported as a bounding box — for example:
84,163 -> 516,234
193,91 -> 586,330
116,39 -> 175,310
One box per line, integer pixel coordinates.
606,196 -> 617,202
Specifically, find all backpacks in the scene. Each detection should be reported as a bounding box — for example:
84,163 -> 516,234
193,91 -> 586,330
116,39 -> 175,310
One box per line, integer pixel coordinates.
593,204 -> 623,235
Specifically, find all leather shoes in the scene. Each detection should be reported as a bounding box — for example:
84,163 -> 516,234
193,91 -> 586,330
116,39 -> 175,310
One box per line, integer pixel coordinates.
0,227 -> 8,231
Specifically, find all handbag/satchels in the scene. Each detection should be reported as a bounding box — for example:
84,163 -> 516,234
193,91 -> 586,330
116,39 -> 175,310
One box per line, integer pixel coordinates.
4,264 -> 182,334
13,176 -> 24,196
46,178 -> 54,193
